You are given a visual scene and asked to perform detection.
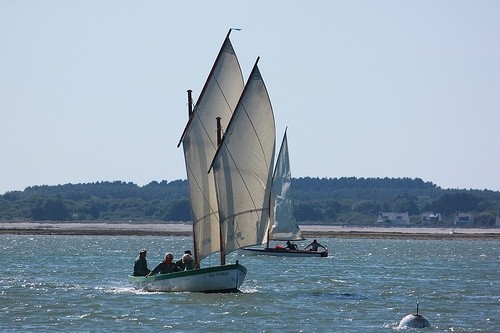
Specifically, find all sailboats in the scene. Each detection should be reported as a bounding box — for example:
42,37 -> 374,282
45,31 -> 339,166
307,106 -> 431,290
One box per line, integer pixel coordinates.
240,125 -> 328,258
125,24 -> 278,293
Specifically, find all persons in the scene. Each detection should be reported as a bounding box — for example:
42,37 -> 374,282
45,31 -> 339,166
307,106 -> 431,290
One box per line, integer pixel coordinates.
177,250 -> 193,271
182,254 -> 200,271
284,241 -> 298,251
148,253 -> 183,276
304,238 -> 325,251
133,249 -> 151,276
